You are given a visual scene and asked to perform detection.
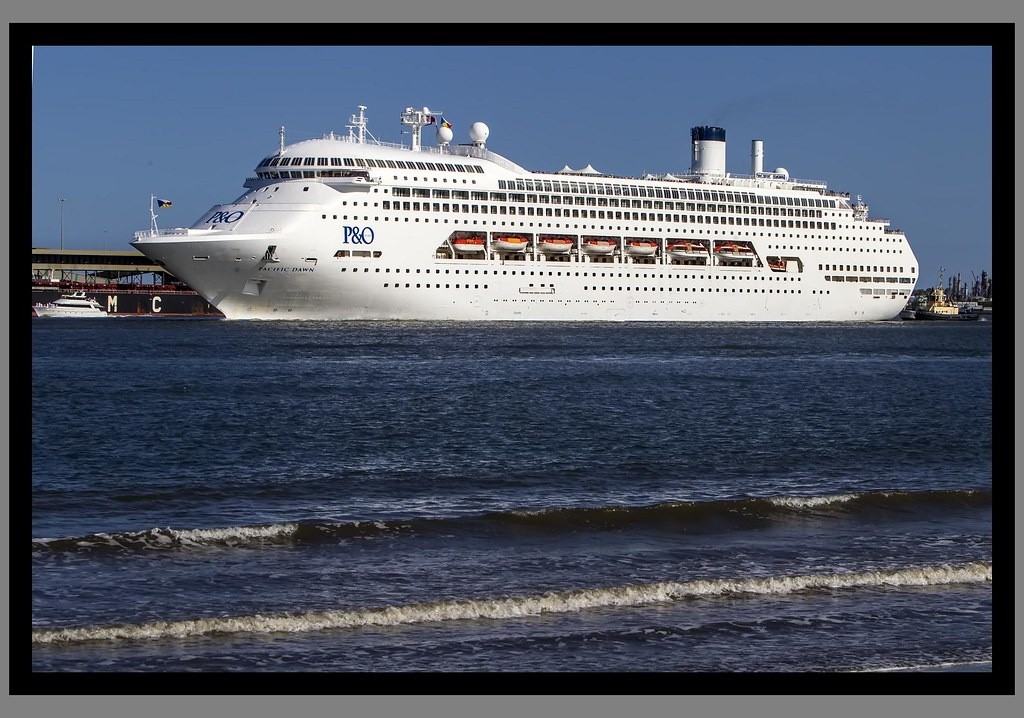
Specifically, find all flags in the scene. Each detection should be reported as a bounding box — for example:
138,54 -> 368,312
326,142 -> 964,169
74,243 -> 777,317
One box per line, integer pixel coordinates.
157,199 -> 173,207
441,116 -> 452,129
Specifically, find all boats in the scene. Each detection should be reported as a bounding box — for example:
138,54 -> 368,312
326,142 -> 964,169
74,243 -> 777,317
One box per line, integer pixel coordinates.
128,104 -> 921,324
899,310 -> 917,320
32,292 -> 109,318
916,287 -> 985,321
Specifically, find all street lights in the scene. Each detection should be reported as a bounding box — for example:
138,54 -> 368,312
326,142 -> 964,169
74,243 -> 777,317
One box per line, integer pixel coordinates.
56,198 -> 67,249
